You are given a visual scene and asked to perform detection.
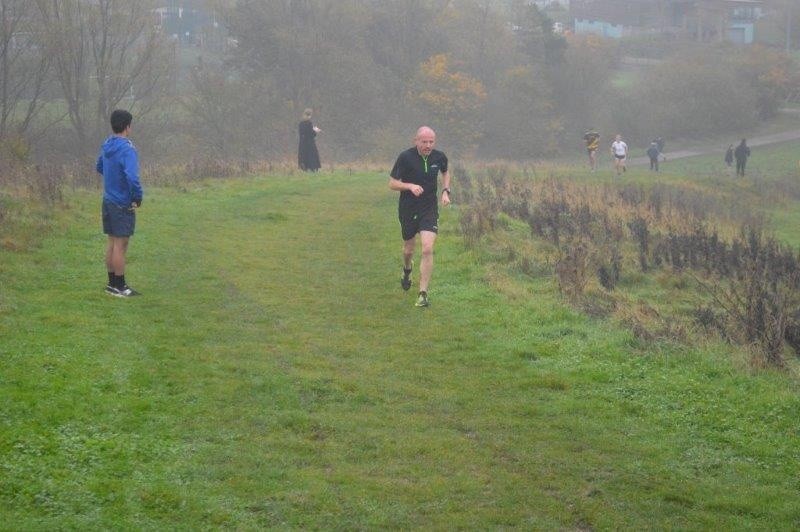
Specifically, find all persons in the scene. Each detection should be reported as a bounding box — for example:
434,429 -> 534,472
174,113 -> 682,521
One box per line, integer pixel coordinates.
297,108 -> 322,171
647,135 -> 751,179
96,110 -> 142,299
612,136 -> 628,174
388,125 -> 451,308
583,127 -> 600,173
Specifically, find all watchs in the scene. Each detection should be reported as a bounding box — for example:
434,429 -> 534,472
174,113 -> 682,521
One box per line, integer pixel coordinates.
442,187 -> 450,196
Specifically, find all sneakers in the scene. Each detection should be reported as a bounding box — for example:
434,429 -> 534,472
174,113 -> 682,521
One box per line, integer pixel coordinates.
105,284 -> 142,298
415,290 -> 429,307
401,259 -> 414,292
